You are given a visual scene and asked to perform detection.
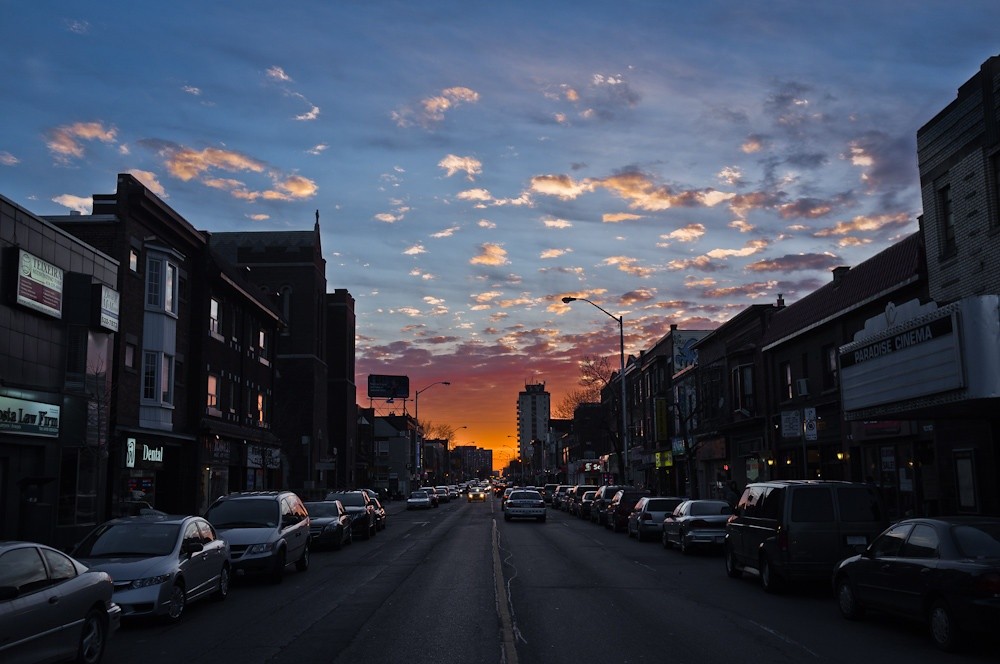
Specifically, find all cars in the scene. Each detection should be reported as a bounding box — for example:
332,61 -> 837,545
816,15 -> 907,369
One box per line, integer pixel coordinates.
368,497 -> 387,530
69,507 -> 233,624
626,496 -> 684,543
604,489 -> 652,534
661,499 -> 735,555
302,499 -> 353,552
833,515 -> 1000,653
407,475 -> 605,521
0,538 -> 122,664
503,490 -> 547,523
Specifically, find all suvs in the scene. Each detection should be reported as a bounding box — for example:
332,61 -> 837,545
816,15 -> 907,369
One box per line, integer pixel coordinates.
321,490 -> 378,540
201,489 -> 312,584
589,485 -> 637,525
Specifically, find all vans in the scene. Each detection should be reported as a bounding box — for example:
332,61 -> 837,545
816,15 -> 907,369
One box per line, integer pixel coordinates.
723,478 -> 882,594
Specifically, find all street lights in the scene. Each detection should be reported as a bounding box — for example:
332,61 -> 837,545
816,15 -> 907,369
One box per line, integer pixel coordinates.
561,296 -> 629,486
415,381 -> 451,490
449,426 -> 468,481
